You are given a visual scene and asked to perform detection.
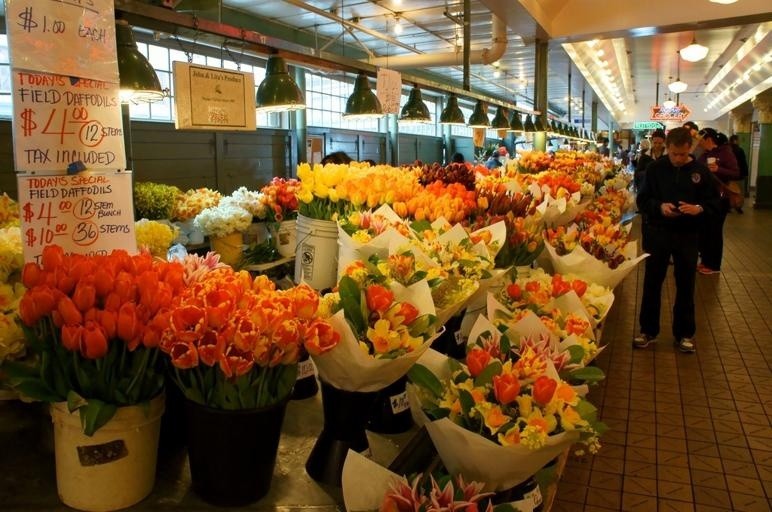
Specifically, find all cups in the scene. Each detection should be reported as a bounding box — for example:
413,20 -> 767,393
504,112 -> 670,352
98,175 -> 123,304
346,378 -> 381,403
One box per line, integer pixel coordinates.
706,157 -> 717,168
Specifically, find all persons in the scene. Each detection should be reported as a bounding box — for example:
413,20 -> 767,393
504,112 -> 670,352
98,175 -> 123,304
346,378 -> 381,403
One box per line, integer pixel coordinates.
572,120 -> 751,275
630,127 -> 722,353
482,144 -> 514,169
449,152 -> 464,164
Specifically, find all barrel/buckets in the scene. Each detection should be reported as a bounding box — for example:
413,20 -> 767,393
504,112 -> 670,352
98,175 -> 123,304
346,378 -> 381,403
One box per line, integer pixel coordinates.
295,210 -> 342,289
261,217 -> 302,260
46,389 -> 168,507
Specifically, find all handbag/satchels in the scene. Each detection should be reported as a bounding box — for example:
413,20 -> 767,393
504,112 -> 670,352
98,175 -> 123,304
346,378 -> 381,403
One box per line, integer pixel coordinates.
726,180 -> 744,208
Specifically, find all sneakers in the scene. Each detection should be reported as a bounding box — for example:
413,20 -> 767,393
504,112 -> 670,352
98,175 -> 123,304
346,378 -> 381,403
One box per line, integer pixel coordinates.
634,333 -> 656,348
696,264 -> 707,270
700,268 -> 721,274
674,337 -> 695,352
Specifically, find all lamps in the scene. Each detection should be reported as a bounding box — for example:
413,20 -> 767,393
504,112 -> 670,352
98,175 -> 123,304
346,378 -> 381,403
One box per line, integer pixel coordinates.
254,46 -> 308,114
108,19 -> 166,107
342,70 -> 544,134
